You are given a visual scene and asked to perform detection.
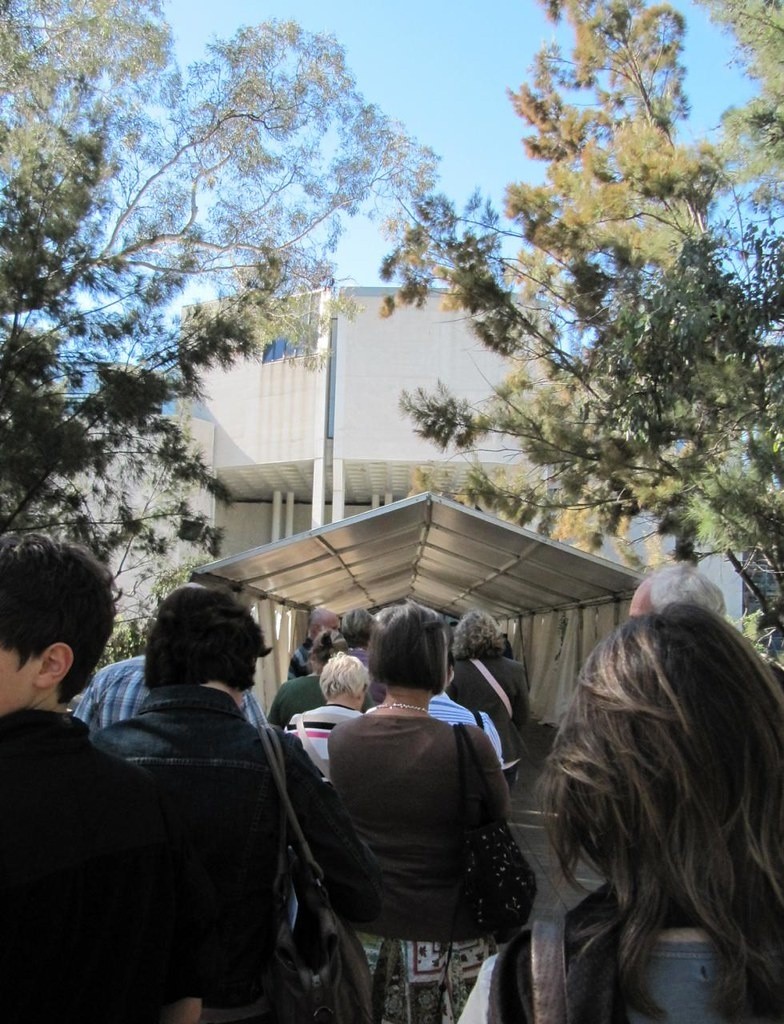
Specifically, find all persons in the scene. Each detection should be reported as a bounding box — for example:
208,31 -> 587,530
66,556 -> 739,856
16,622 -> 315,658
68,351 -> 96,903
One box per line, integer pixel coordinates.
0,536 -> 205,1024
460,604 -> 783,1024
72,583 -> 530,1024
629,565 -> 725,618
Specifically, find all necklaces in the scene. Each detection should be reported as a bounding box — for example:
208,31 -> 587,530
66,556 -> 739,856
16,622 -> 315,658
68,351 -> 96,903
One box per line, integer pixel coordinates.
366,702 -> 428,714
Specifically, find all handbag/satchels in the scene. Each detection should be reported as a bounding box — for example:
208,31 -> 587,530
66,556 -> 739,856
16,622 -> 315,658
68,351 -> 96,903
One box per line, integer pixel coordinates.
260,727 -> 374,1024
435,723 -> 536,942
